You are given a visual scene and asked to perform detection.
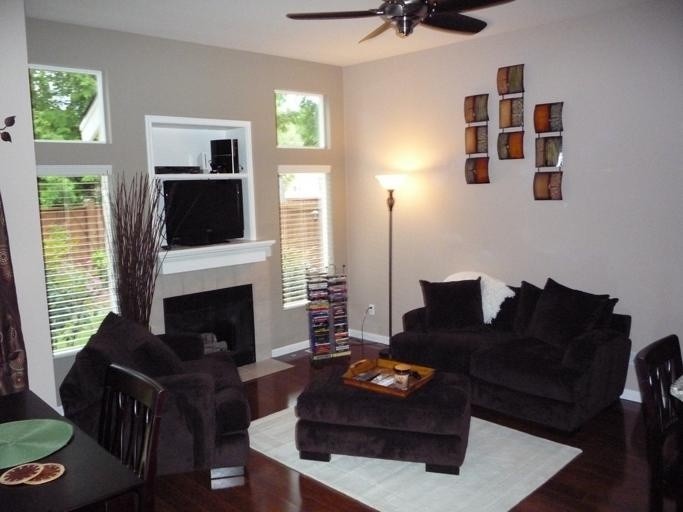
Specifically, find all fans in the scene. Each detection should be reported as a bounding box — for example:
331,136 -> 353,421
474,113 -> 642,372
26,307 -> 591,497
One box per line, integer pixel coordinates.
286,0 -> 514,43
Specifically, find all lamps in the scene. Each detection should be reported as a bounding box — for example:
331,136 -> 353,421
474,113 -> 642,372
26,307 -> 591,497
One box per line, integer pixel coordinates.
373,174 -> 404,359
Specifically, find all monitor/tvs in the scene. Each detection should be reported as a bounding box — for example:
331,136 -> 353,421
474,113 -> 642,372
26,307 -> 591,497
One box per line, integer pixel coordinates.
162,179 -> 245,246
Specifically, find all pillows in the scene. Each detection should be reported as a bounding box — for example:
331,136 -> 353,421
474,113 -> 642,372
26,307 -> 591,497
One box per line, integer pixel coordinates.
525,277 -> 609,354
419,277 -> 486,334
97,312 -> 184,376
512,281 -> 543,330
593,298 -> 619,340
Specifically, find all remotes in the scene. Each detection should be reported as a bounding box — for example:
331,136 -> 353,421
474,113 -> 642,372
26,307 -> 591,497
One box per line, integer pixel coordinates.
365,373 -> 380,380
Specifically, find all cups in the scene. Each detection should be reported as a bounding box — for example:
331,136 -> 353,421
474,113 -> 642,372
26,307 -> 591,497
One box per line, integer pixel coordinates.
394,364 -> 410,390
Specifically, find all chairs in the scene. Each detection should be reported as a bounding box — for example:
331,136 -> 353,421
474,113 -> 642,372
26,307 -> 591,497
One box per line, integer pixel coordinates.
97,362 -> 165,512
633,334 -> 683,512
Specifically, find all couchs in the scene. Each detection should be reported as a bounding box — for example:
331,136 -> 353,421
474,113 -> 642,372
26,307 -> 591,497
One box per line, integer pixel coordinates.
60,332 -> 250,485
390,307 -> 631,432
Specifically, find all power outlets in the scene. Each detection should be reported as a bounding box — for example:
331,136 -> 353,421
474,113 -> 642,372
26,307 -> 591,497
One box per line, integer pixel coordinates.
368,304 -> 376,315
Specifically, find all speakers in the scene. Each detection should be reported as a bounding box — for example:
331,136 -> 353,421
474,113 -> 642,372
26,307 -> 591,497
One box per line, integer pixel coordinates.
210,138 -> 239,173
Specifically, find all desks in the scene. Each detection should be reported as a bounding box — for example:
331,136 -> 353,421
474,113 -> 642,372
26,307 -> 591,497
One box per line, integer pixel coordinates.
294,364 -> 472,475
0,391 -> 145,512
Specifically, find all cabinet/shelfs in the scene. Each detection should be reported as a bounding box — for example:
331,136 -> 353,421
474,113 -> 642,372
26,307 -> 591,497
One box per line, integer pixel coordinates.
305,264 -> 352,370
